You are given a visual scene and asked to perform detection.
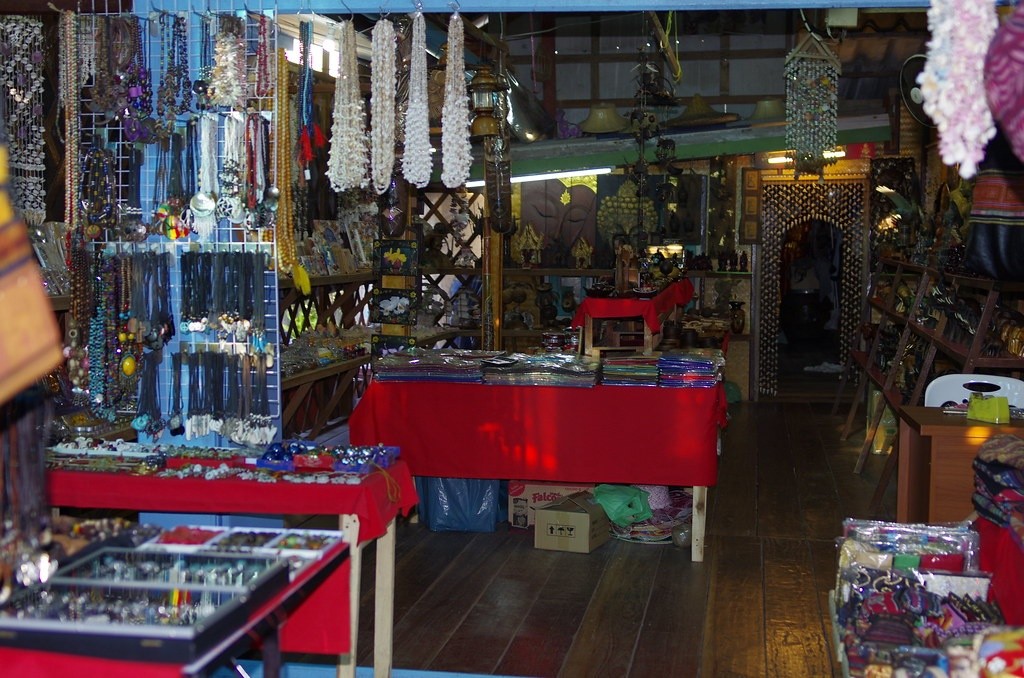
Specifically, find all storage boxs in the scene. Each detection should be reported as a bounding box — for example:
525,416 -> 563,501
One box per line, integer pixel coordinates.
507,480 -> 595,529
867,379 -> 898,455
535,490 -> 610,553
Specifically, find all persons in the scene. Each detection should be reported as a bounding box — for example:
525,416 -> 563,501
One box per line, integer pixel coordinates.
448,274 -> 482,347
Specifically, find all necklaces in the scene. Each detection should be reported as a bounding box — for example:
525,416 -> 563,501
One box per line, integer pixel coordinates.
345,460 -> 400,502
275,14 -> 474,272
0,387 -> 59,589
59,10 -> 278,446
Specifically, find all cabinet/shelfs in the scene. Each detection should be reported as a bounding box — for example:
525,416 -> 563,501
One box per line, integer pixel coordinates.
275,52 -> 505,442
705,270 -> 752,341
583,277 -> 695,356
896,405 -> 1023,524
834,260 -> 1024,519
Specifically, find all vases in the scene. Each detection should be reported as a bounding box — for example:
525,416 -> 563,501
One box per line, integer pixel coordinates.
729,302 -> 746,334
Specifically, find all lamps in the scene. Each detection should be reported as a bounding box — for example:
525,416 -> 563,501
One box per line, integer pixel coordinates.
467,64 -> 508,137
464,165 -> 616,189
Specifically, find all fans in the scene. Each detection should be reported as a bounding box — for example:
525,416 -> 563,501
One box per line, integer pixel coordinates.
899,54 -> 937,129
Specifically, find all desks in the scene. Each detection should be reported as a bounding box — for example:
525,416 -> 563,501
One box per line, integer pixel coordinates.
350,348 -> 729,563
1,440 -> 421,678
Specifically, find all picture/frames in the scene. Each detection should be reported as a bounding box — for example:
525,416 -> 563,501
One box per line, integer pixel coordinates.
371,239 -> 419,276
369,288 -> 419,326
370,334 -> 416,372
740,168 -> 762,244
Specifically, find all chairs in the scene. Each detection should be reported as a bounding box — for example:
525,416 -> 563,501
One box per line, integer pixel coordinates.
925,374 -> 1024,409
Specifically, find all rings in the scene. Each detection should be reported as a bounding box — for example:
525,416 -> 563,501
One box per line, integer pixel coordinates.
166,444 -> 232,460
